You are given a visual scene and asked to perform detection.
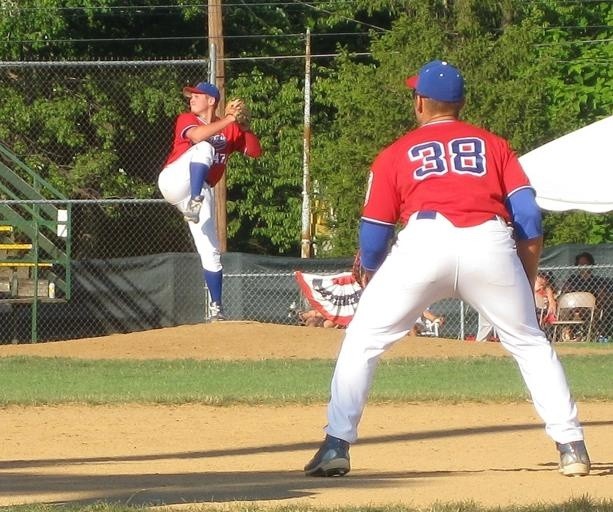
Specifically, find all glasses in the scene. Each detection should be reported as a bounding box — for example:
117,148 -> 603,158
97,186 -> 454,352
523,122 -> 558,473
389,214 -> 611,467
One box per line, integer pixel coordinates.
411,89 -> 430,100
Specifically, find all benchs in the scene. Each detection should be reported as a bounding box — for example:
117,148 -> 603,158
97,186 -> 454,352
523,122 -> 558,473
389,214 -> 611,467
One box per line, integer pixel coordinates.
1,227 -> 66,344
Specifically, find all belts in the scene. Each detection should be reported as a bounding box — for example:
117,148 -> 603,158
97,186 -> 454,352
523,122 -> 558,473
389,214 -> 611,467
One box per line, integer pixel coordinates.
416,210 -> 497,220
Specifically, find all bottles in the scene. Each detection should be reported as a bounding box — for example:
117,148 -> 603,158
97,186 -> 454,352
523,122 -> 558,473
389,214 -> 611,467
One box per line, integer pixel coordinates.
598,335 -> 608,343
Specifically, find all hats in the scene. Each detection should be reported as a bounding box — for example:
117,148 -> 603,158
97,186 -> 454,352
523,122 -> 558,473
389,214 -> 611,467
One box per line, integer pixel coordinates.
404,58 -> 465,103
181,81 -> 221,101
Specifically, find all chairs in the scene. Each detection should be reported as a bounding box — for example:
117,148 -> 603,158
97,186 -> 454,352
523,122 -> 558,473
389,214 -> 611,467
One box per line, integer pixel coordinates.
418,320 -> 439,337
493,292 -> 547,339
550,291 -> 595,342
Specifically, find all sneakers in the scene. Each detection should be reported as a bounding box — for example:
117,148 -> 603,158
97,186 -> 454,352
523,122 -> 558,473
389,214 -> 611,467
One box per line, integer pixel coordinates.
554,438 -> 591,478
303,434 -> 351,478
181,194 -> 204,224
207,301 -> 226,322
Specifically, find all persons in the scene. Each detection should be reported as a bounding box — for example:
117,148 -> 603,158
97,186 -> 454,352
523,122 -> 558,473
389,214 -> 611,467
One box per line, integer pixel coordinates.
561,250 -> 612,344
412,311 -> 446,337
157,80 -> 264,323
297,306 -> 344,330
303,61 -> 593,478
533,270 -> 558,332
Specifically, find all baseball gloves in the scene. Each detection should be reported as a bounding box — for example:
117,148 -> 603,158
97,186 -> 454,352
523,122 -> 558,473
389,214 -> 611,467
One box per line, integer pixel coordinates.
224,98 -> 252,127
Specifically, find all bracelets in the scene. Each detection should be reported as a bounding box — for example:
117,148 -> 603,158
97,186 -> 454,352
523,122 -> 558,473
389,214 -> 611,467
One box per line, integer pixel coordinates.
224,112 -> 235,118
